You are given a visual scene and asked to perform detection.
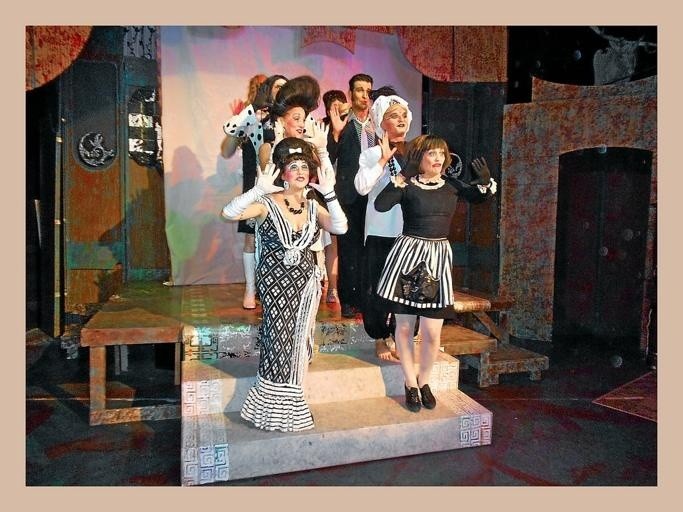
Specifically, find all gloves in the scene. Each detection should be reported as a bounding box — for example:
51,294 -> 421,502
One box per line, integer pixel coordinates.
308,161 -> 348,236
469,156 -> 492,186
270,120 -> 284,163
304,120 -> 337,185
397,148 -> 426,180
222,163 -> 284,218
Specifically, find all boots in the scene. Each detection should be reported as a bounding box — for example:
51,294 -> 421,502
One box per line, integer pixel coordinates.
242,252 -> 257,309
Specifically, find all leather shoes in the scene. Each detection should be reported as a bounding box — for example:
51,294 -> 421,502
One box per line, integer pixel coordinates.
416,374 -> 437,410
404,381 -> 421,413
341,304 -> 356,318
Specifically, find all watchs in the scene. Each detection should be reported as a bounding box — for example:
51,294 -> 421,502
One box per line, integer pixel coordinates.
221,137 -> 348,434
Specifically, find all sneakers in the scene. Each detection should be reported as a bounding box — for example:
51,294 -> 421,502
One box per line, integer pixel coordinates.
326,288 -> 337,303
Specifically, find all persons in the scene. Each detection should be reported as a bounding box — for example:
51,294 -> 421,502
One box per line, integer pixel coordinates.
352,95 -> 416,361
372,132 -> 498,412
221,71 -> 396,320
